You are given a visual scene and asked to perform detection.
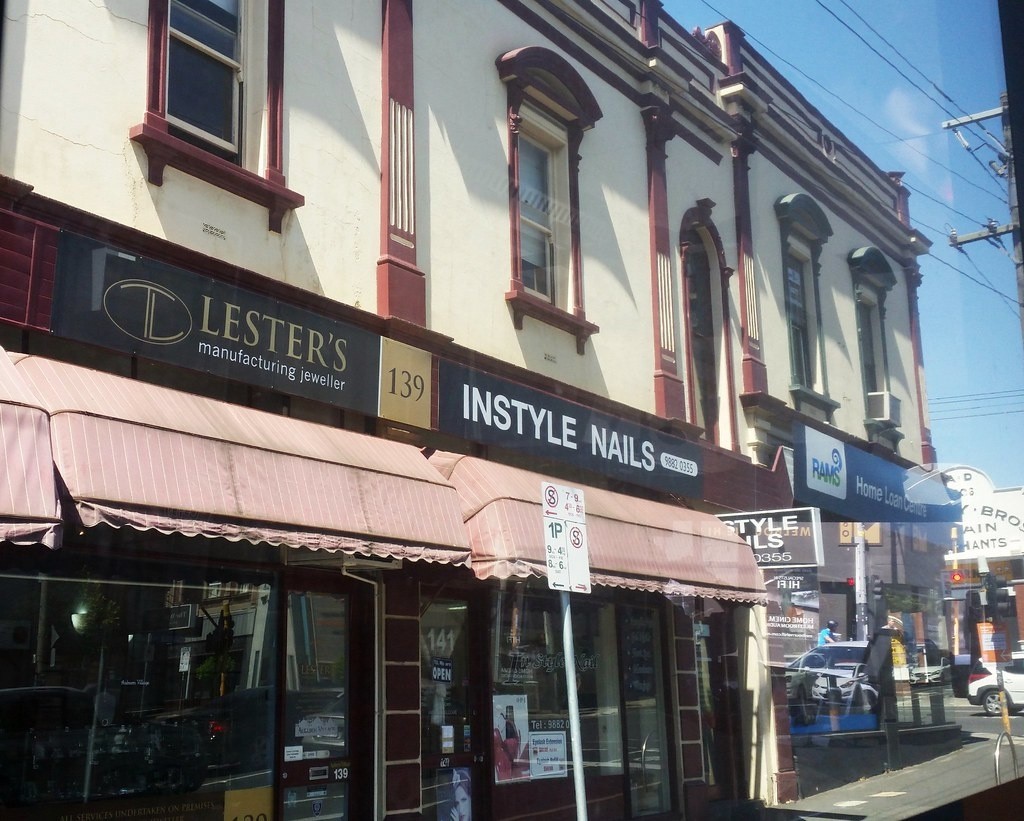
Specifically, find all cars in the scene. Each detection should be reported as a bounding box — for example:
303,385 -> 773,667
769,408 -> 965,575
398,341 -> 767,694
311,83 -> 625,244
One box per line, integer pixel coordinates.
155,685 -> 349,767
908,657 -> 951,684
778,640 -> 884,698
811,661 -> 869,703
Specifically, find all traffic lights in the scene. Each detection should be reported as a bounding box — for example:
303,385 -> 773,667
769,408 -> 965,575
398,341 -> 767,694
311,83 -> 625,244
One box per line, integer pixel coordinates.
847,577 -> 855,593
953,572 -> 963,582
870,574 -> 884,604
989,573 -> 1011,623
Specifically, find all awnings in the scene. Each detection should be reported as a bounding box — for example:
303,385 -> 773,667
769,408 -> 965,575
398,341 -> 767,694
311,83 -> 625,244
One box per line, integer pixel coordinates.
0,345 -> 63,550
430,450 -> 772,605
18,350 -> 468,568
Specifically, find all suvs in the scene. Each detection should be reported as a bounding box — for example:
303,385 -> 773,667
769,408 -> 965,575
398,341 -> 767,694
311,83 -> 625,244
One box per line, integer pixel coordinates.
967,651 -> 1024,716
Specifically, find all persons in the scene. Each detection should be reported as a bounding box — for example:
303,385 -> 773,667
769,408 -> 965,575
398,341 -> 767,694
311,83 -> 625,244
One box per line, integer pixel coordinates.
818,621 -> 838,646
882,618 -> 897,629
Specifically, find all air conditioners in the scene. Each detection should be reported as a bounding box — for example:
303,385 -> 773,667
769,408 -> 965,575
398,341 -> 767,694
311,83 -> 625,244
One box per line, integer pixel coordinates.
868,392 -> 902,428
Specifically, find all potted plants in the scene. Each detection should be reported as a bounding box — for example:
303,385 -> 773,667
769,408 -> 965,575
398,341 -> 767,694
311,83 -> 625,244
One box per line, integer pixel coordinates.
69,571 -> 123,638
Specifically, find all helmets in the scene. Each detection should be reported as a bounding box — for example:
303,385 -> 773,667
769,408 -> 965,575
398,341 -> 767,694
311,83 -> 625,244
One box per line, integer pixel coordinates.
828,620 -> 838,628
